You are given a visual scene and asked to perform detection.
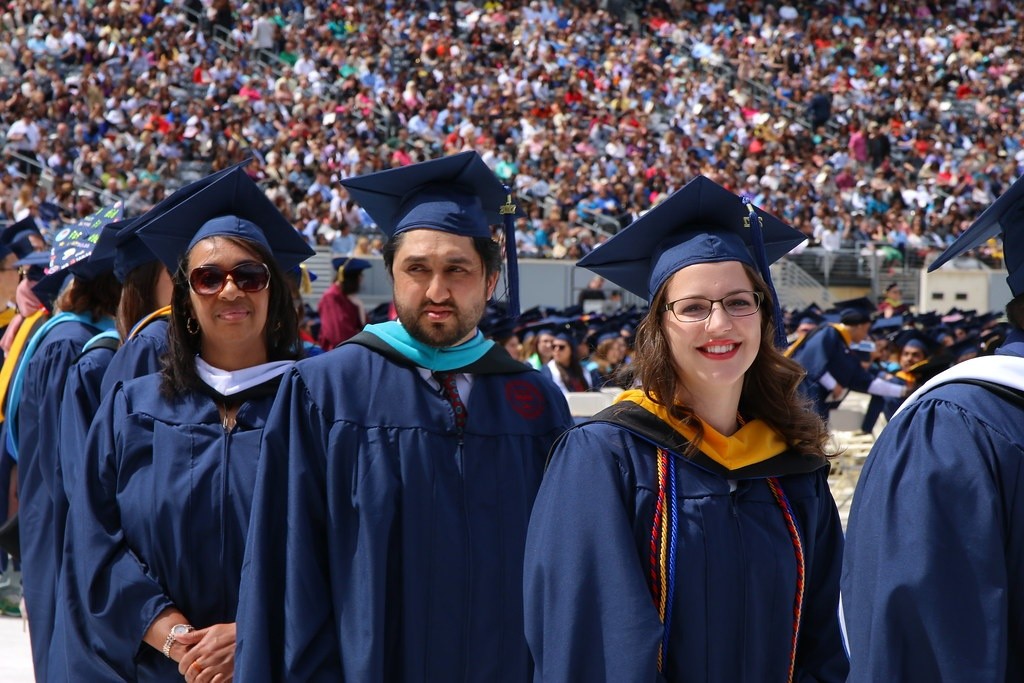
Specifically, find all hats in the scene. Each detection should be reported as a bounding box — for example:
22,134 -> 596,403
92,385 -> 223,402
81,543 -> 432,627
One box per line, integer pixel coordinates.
338,151 -> 527,321
0,200 -> 317,314
927,174 -> 1024,298
481,282 -> 1009,360
576,174 -> 809,350
135,167 -> 317,296
332,256 -> 372,286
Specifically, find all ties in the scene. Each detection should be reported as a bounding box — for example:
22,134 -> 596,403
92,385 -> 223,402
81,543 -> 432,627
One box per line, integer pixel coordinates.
434,369 -> 468,447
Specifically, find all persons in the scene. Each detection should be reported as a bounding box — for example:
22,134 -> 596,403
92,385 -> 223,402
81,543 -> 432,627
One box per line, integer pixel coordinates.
58,167 -> 315,683
779,282 -> 1013,431
479,275 -> 651,393
839,172 -> 1024,683
295,257 -> 398,355
0,1 -> 1024,259
0,199 -> 170,683
234,152 -> 575,683
521,174 -> 851,683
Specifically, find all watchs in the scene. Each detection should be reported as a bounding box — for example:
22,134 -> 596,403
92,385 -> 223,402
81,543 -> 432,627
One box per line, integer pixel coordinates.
163,624 -> 194,658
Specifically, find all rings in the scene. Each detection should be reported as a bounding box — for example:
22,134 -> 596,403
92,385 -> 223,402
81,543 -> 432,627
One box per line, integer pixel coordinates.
192,661 -> 202,673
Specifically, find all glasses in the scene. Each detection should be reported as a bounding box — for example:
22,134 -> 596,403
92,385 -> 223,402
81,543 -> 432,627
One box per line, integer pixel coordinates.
657,290 -> 765,322
186,262 -> 271,295
